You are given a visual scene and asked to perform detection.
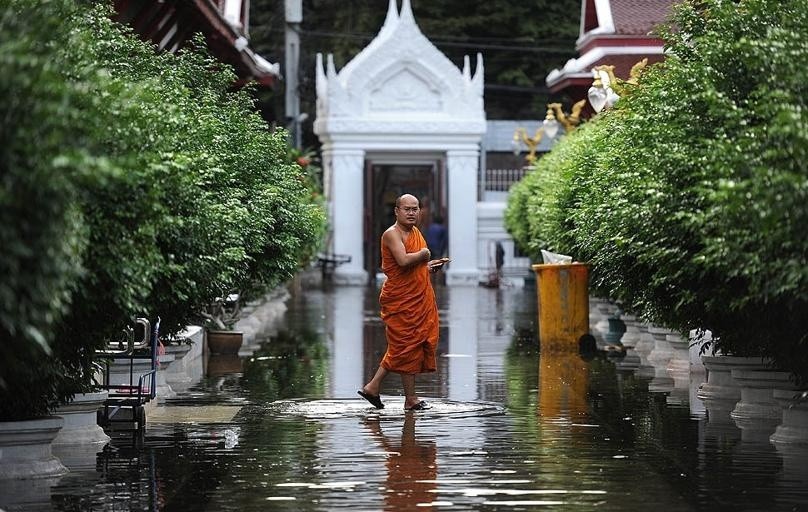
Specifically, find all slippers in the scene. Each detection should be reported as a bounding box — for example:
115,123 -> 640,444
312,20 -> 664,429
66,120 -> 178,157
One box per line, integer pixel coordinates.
357,390 -> 385,409
403,400 -> 435,411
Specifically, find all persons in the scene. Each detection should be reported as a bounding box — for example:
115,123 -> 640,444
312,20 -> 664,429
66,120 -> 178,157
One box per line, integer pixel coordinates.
419,195 -> 431,229
423,214 -> 448,284
356,408 -> 441,512
357,192 -> 450,410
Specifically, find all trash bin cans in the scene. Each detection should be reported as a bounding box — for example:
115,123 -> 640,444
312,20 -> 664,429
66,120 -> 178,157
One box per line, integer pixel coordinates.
531,262 -> 596,352
